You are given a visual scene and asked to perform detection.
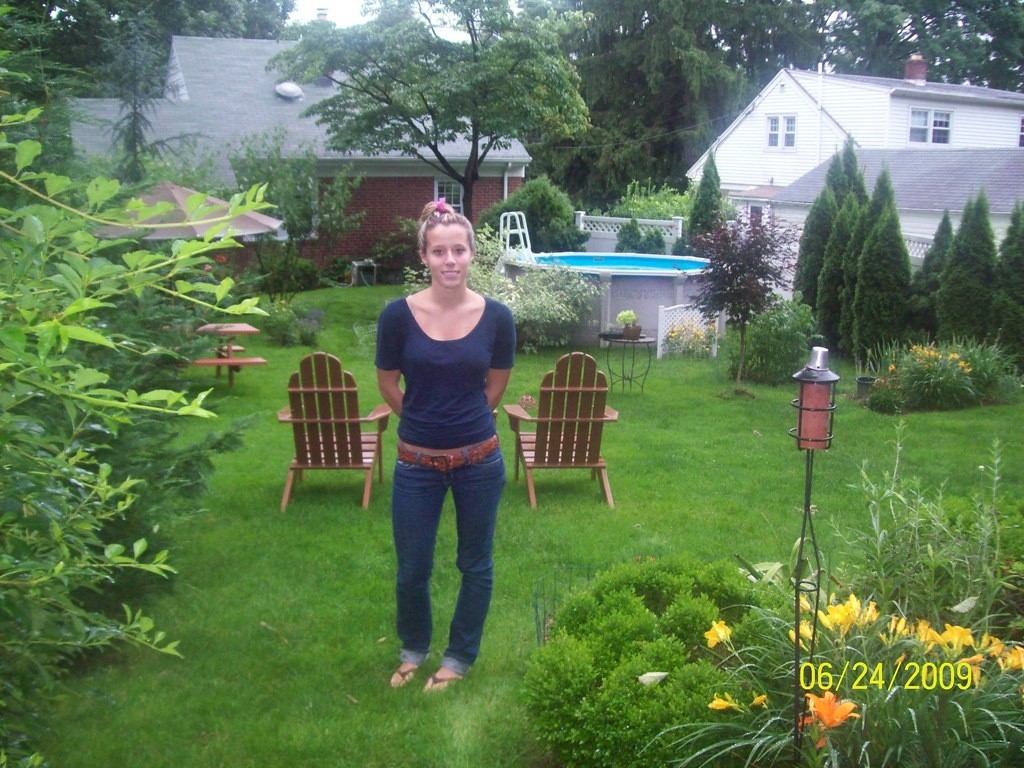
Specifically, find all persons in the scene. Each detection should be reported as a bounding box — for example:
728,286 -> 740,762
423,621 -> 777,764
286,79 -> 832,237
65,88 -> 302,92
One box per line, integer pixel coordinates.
373,201 -> 516,690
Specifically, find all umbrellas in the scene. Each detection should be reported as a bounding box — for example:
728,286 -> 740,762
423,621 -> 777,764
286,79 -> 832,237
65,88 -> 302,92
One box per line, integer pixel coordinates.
93,181 -> 283,241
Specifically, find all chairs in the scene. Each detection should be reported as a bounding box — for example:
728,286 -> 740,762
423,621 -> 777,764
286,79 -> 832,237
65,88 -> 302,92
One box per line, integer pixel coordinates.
276,351 -> 394,512
502,351 -> 619,510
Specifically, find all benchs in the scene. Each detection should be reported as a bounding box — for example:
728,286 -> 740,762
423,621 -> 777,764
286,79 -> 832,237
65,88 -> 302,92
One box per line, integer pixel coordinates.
191,345 -> 267,373
187,344 -> 244,354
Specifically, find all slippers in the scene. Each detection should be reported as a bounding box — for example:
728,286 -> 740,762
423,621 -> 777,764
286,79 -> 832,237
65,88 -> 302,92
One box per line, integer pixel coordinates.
391,668 -> 418,688
424,674 -> 464,696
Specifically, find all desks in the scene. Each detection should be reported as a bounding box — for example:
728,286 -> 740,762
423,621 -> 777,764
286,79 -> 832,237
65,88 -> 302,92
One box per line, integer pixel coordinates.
193,323 -> 260,387
603,337 -> 656,396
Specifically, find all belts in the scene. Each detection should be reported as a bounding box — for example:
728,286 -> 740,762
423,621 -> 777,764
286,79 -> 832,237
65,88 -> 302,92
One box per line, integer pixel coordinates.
398,434 -> 500,472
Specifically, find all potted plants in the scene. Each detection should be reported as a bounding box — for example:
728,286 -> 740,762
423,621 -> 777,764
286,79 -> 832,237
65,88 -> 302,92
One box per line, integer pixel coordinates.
616,309 -> 642,340
